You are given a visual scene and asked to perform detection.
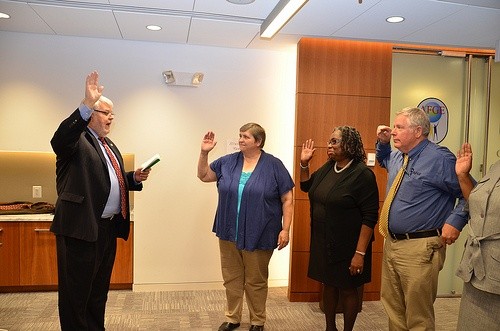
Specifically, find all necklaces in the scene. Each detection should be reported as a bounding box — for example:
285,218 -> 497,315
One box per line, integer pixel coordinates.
334,159 -> 354,173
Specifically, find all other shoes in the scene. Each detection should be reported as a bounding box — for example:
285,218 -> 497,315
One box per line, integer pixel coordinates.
249,324 -> 264,331
218,322 -> 240,331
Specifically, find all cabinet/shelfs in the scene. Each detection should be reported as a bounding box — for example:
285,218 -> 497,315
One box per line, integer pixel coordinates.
110,221 -> 134,283
0,222 -> 20,287
20,221 -> 60,286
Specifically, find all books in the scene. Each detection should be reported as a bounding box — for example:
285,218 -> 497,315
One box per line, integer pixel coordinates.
141,155 -> 160,172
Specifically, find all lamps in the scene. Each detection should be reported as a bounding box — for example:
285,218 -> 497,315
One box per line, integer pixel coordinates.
260,0 -> 309,39
163,70 -> 204,87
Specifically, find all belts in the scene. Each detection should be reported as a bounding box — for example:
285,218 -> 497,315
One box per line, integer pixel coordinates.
391,229 -> 442,240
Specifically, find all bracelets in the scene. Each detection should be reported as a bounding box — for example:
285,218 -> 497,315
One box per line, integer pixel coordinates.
300,162 -> 309,168
355,250 -> 366,255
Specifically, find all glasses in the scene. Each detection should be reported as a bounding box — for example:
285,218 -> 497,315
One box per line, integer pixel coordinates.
327,138 -> 342,145
94,109 -> 115,116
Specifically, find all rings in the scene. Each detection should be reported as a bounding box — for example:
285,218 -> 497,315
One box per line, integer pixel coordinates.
357,269 -> 360,273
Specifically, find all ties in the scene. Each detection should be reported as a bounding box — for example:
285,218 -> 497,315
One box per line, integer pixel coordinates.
378,155 -> 410,240
98,137 -> 127,219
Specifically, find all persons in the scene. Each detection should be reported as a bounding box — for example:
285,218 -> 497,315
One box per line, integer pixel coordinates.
51,71 -> 151,331
299,126 -> 379,331
199,122 -> 294,331
375,107 -> 500,331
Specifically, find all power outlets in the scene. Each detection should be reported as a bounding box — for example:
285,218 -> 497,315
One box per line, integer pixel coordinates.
33,186 -> 42,198
